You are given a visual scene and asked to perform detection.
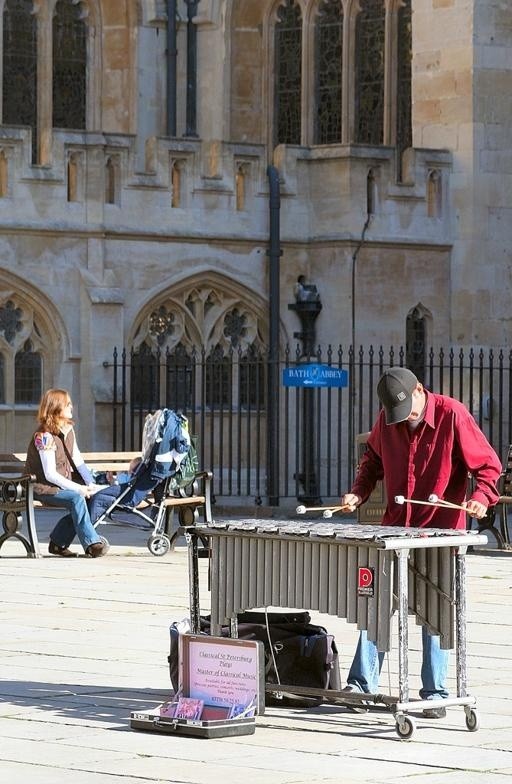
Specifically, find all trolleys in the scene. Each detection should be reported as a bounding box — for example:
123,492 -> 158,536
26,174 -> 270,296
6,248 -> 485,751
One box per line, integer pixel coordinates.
168,523 -> 487,739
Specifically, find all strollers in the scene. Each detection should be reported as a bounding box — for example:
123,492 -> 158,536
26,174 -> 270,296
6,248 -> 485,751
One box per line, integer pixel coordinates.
85,407 -> 213,555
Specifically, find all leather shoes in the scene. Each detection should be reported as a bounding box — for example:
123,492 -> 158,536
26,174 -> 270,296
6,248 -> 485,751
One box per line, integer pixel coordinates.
327,685 -> 366,713
423,707 -> 445,718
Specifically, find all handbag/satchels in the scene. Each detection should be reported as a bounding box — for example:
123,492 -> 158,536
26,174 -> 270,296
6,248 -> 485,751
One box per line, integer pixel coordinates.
168,611 -> 341,709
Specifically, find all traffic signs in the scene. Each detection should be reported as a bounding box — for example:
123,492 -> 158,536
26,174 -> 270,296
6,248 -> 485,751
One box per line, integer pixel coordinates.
282,364 -> 348,387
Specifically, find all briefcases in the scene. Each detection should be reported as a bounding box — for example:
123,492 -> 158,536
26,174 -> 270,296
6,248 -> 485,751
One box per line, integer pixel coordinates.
129,633 -> 265,738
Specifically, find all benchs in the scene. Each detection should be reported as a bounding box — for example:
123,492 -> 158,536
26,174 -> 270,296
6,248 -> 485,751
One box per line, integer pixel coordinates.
0,450 -> 216,558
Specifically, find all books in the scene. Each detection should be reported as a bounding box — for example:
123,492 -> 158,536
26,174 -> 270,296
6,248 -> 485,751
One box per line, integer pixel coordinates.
159,695 -> 255,720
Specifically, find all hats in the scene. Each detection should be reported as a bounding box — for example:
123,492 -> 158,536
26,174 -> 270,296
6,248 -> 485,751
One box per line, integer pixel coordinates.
377,367 -> 418,425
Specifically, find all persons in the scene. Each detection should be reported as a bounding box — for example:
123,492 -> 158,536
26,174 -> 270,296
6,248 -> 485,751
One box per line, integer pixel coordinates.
106,456 -> 149,508
20,387 -> 110,558
338,368 -> 503,718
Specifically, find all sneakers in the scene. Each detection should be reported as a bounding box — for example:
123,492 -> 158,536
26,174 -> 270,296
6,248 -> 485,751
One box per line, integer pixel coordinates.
49,543 -> 76,557
92,544 -> 106,556
